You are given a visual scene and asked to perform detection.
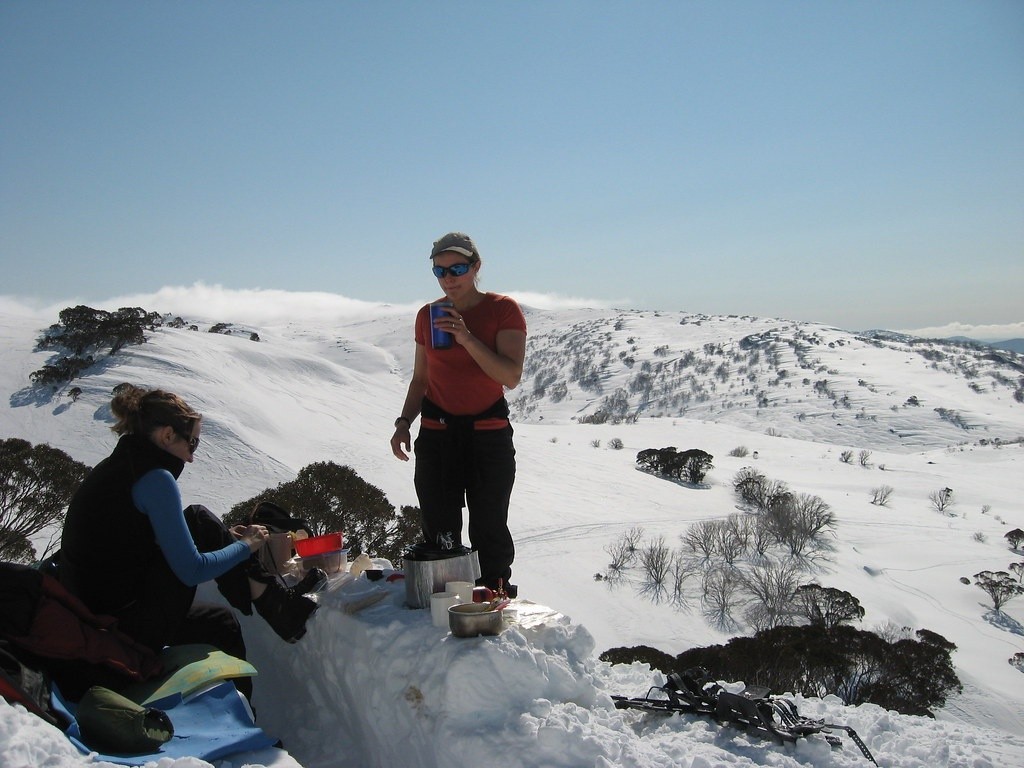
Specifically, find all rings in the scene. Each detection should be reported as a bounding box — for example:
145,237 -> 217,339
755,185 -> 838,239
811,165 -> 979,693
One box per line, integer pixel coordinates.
453,324 -> 455,328
459,318 -> 462,322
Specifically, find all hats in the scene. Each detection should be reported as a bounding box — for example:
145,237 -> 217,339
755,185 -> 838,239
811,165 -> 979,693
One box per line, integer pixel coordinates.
430,233 -> 479,259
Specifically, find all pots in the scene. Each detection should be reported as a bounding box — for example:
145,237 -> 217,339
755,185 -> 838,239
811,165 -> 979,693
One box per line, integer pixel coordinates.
402,532 -> 483,608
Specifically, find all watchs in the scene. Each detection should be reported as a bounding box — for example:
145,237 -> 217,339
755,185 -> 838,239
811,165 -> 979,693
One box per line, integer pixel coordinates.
394,417 -> 411,429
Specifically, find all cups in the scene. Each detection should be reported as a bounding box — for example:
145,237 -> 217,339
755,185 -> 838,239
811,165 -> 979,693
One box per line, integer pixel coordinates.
431,592 -> 458,627
262,533 -> 292,571
430,302 -> 453,350
445,581 -> 473,603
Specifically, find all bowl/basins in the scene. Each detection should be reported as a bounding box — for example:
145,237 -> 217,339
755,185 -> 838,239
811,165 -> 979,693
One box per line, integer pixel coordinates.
350,553 -> 373,577
296,549 -> 348,578
294,533 -> 343,557
449,604 -> 501,636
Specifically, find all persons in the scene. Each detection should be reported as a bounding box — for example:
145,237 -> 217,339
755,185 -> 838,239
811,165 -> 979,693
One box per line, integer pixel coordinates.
56,382 -> 329,749
389,232 -> 528,598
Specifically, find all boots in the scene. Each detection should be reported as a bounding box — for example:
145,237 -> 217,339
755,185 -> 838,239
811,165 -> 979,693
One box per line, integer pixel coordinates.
256,568 -> 328,645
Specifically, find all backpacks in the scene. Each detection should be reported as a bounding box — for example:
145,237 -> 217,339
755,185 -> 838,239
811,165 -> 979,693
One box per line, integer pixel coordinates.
245,502 -> 314,558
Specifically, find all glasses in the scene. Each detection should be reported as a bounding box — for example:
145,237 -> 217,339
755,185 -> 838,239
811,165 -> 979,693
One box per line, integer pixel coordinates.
432,260 -> 476,278
154,420 -> 200,455
